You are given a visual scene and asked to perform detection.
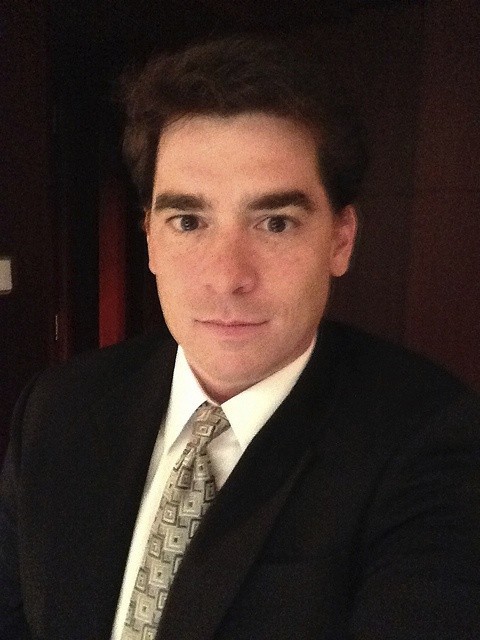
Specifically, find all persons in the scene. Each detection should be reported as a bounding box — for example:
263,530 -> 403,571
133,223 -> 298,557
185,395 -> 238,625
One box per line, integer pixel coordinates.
1,39 -> 479,639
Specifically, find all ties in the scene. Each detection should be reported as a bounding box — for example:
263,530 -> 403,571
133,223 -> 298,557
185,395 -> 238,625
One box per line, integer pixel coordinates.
122,403 -> 231,640
